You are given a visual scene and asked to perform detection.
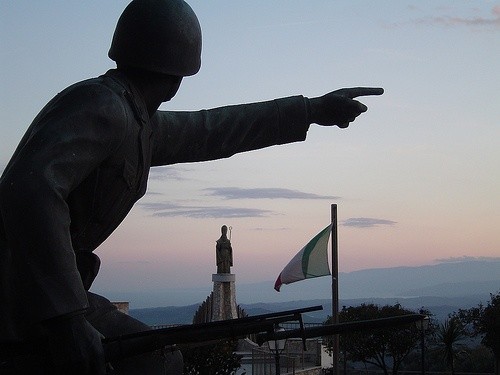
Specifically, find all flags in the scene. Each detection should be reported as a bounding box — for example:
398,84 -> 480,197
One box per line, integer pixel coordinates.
274,222 -> 332,293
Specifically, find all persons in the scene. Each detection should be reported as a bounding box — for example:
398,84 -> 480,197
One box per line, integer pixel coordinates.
216,223 -> 235,274
1,0 -> 387,375
